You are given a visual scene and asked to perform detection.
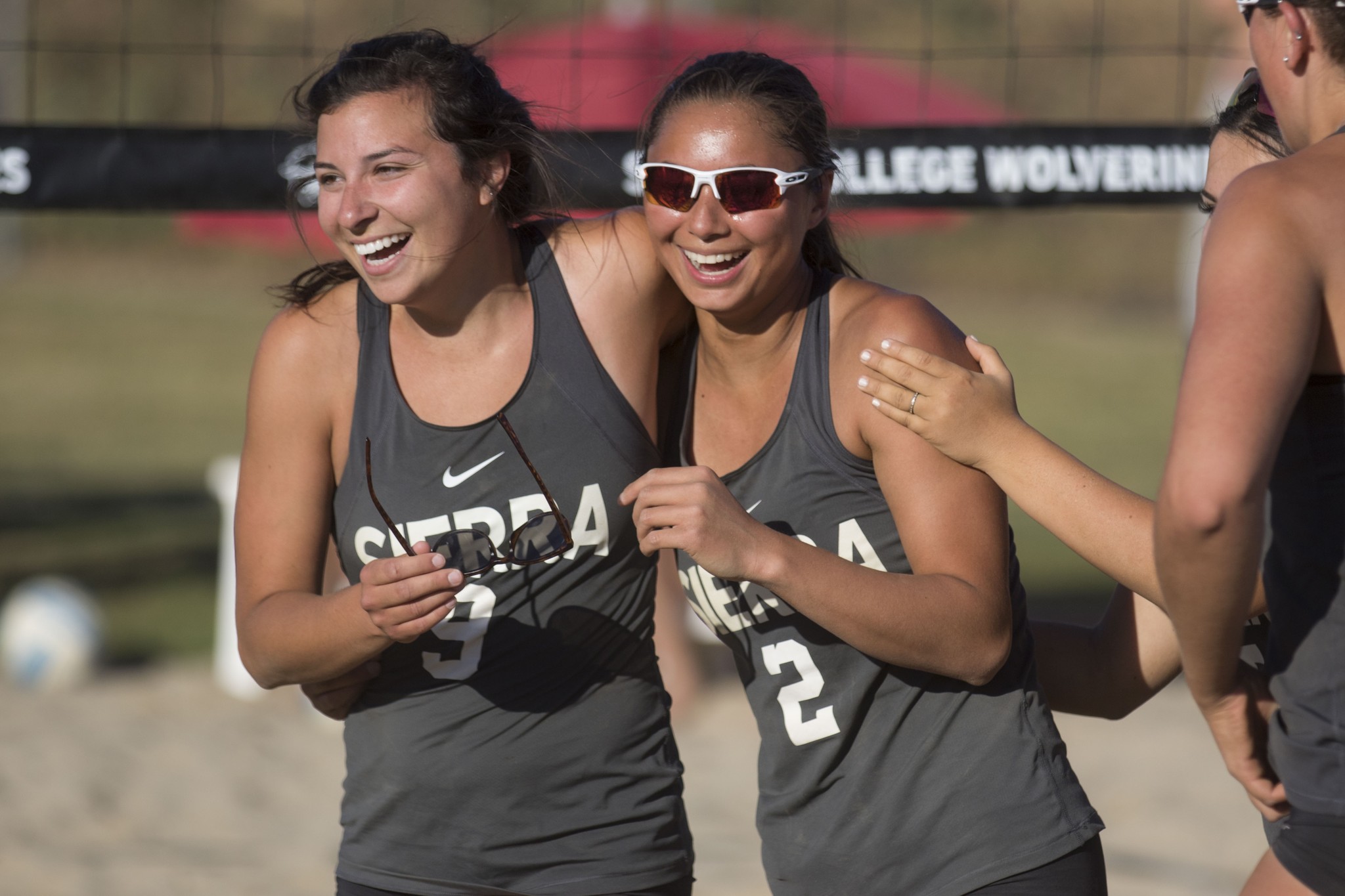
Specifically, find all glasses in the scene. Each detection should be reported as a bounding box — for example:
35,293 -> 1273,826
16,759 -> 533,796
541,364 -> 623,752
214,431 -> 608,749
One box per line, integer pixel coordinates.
1235,0 -> 1345,28
366,414 -> 575,577
634,162 -> 824,215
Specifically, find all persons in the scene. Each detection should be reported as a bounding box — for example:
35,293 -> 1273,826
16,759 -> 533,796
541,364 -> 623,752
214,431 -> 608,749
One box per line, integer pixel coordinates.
296,49 -> 1110,896
233,27 -> 693,896
1152,0 -> 1345,896
857,65 -> 1288,721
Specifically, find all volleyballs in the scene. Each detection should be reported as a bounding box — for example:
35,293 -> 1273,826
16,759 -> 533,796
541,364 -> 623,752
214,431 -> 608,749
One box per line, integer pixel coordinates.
0,575 -> 102,691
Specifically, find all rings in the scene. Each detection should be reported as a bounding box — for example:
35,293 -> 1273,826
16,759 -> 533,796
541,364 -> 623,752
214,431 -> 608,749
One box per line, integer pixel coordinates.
908,390 -> 919,412
